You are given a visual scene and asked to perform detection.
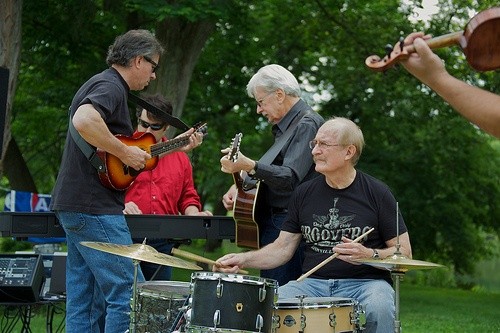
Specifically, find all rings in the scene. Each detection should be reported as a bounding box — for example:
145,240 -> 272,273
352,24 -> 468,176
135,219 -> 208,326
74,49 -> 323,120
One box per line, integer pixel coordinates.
349,256 -> 352,261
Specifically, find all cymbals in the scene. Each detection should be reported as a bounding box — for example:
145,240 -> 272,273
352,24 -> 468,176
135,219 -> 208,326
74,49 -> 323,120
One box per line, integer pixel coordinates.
348,254 -> 448,271
77,238 -> 203,272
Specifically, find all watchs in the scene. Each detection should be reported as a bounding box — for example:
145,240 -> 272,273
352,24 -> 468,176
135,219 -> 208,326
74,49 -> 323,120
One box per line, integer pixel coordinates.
247,160 -> 258,180
372,248 -> 379,259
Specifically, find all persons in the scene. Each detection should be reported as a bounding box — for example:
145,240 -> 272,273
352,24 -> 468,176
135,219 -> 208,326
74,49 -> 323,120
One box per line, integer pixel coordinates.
212,116 -> 412,333
49,31 -> 203,333
220,64 -> 326,290
121,96 -> 213,281
393,31 -> 500,139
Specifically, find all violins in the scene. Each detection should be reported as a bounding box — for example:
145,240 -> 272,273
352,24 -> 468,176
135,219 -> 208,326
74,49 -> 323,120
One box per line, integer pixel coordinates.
364,6 -> 500,79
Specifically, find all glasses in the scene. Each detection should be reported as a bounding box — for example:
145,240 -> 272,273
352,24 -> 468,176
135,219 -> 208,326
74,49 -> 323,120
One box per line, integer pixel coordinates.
309,139 -> 352,150
139,119 -> 165,131
255,90 -> 277,107
144,55 -> 160,73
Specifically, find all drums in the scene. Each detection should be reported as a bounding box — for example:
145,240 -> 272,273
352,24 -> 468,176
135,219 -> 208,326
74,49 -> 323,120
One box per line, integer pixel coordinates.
273,296 -> 360,333
130,278 -> 194,333
187,272 -> 278,333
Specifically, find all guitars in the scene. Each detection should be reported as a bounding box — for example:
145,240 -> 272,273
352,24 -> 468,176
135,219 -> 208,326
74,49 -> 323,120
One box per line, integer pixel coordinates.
95,122 -> 210,193
225,133 -> 264,251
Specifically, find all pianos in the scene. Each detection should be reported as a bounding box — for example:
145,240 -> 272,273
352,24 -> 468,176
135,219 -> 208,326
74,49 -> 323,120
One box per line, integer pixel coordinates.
0,210 -> 235,280
